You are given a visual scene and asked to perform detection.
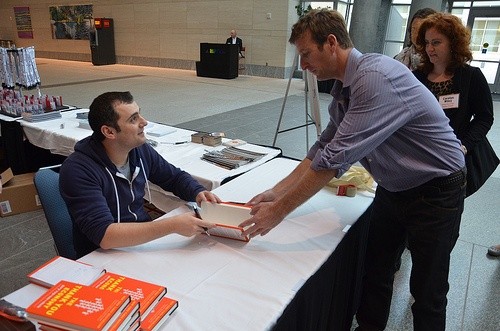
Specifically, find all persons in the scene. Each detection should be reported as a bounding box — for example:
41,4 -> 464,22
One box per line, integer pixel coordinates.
395,12 -> 500,275
238,5 -> 466,331
225,30 -> 243,53
58,92 -> 221,253
488,245 -> 500,257
393,8 -> 439,70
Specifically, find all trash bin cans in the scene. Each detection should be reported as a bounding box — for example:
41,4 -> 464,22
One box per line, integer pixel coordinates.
0,40 -> 13,48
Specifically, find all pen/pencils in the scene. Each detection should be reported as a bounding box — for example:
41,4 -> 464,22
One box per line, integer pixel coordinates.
193,205 -> 210,237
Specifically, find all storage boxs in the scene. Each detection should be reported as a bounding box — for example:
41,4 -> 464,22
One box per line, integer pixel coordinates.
0,167 -> 43,218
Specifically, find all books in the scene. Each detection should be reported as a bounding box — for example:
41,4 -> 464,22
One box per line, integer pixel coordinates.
24,255 -> 179,331
191,132 -> 222,147
200,199 -> 255,242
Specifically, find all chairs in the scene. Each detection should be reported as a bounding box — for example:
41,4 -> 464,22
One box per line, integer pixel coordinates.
34,169 -> 76,260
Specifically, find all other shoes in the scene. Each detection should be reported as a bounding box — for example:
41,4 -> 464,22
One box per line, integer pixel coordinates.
488,244 -> 500,256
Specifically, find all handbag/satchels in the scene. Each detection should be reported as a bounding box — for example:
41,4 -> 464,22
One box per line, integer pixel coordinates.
449,117 -> 500,200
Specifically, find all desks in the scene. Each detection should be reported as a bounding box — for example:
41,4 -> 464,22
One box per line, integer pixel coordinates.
0,104 -> 378,331
